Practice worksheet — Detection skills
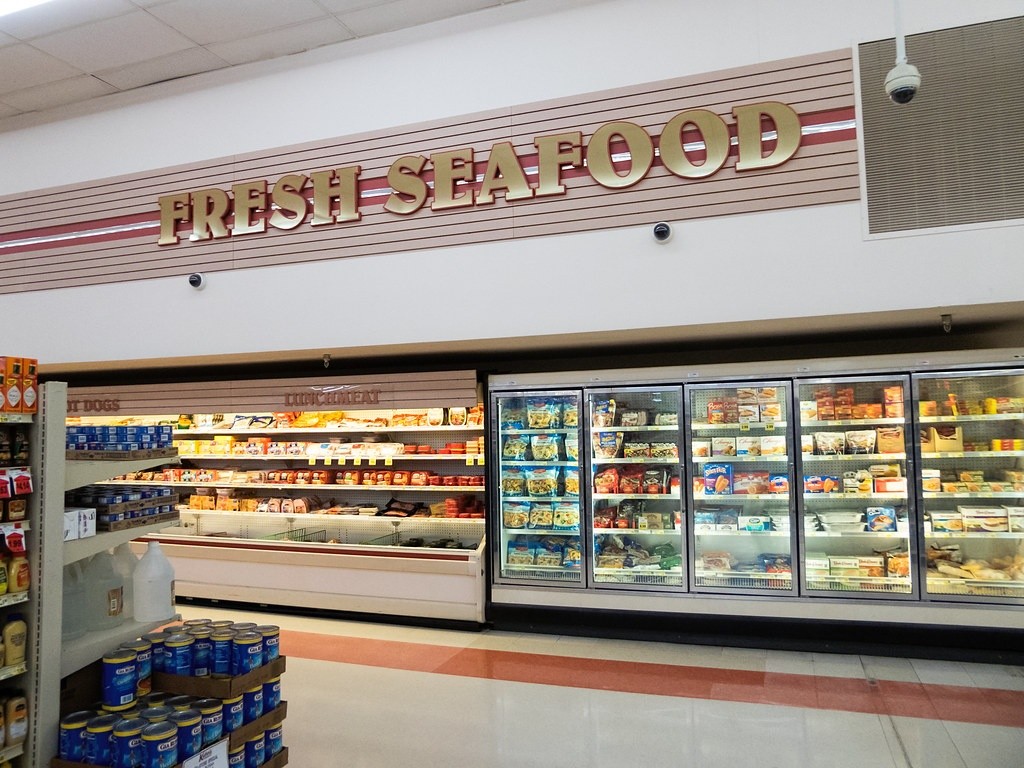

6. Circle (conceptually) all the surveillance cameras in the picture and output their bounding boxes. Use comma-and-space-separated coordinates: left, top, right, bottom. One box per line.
652, 222, 672, 243
188, 272, 206, 290
884, 64, 922, 104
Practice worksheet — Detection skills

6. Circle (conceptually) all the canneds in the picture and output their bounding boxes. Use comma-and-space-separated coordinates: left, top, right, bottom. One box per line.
66, 425, 172, 451
65, 486, 172, 522
60, 619, 283, 768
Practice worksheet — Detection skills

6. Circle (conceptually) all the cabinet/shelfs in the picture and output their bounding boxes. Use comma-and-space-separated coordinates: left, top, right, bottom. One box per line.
0, 381, 183, 768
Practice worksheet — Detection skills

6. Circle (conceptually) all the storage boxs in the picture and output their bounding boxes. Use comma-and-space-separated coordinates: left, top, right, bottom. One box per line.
77, 446, 179, 461
63, 508, 79, 541
95, 510, 180, 531
0, 356, 38, 413
229, 700, 287, 751
163, 468, 180, 482
89, 492, 180, 515
261, 746, 288, 768
150, 653, 287, 701
173, 439, 179, 447
70, 506, 97, 538
64, 424, 174, 450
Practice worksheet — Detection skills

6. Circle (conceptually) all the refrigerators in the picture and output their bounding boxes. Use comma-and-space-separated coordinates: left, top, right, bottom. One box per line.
488, 362, 1024, 611
